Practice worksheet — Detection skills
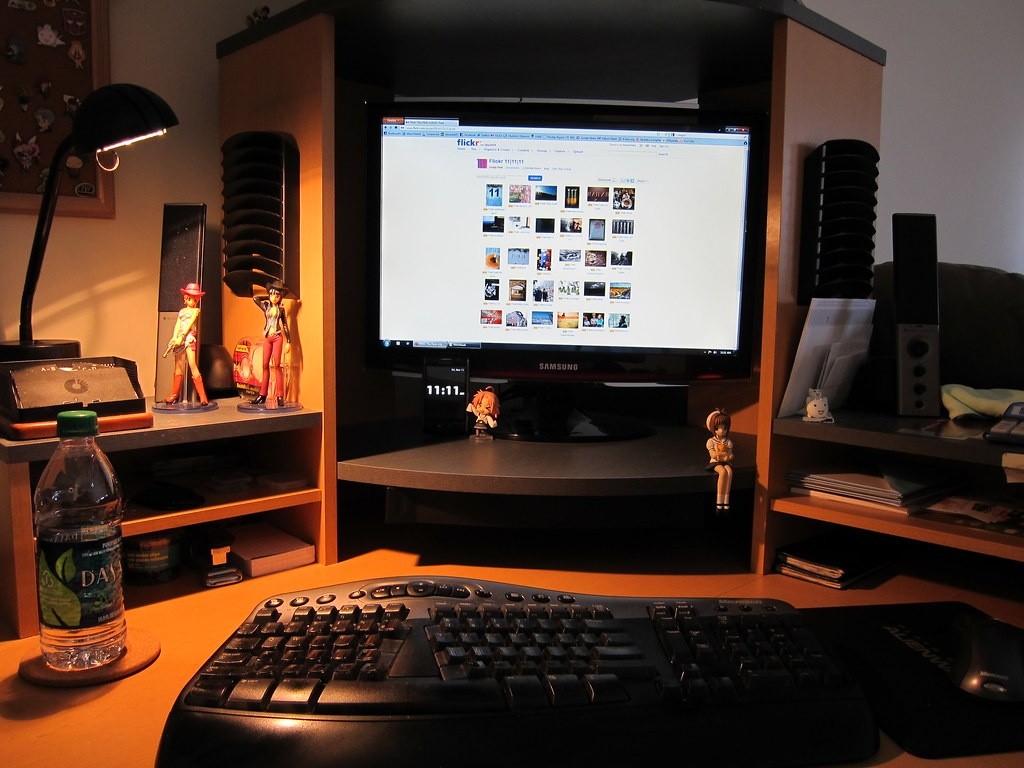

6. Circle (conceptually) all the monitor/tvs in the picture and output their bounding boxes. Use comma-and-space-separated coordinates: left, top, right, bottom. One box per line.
359, 100, 771, 443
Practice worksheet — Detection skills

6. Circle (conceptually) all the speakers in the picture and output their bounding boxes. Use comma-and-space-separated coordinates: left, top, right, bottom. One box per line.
154, 203, 207, 404
893, 213, 941, 418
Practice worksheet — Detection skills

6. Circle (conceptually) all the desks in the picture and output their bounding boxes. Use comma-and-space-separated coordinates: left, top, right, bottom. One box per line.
0, 530, 1024, 768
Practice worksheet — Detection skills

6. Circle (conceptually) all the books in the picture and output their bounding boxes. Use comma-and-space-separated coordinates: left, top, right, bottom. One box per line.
784, 469, 944, 515
773, 536, 893, 589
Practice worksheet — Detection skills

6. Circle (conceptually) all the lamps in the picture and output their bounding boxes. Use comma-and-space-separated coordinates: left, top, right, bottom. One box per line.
0, 80, 178, 362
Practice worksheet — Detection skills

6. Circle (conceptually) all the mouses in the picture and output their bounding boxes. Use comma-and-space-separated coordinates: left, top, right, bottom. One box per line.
939, 659, 1013, 704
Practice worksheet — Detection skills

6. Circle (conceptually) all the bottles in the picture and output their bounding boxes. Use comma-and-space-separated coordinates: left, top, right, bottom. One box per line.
34, 409, 127, 670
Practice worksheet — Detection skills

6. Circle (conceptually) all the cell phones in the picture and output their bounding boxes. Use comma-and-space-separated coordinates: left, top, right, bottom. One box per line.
422, 358, 470, 442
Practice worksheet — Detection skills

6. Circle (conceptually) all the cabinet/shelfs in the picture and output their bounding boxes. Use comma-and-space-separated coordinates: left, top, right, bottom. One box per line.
0, 0, 1024, 633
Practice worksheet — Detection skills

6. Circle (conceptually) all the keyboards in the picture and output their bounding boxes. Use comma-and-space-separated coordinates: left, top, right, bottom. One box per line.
156, 576, 881, 768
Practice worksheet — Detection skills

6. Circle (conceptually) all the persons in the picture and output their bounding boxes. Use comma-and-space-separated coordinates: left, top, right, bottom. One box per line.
249, 285, 291, 407
167, 283, 209, 406
705, 410, 735, 514
465, 391, 499, 437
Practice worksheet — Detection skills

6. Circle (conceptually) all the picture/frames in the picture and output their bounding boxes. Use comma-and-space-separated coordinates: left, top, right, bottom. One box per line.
0, 0, 116, 220
